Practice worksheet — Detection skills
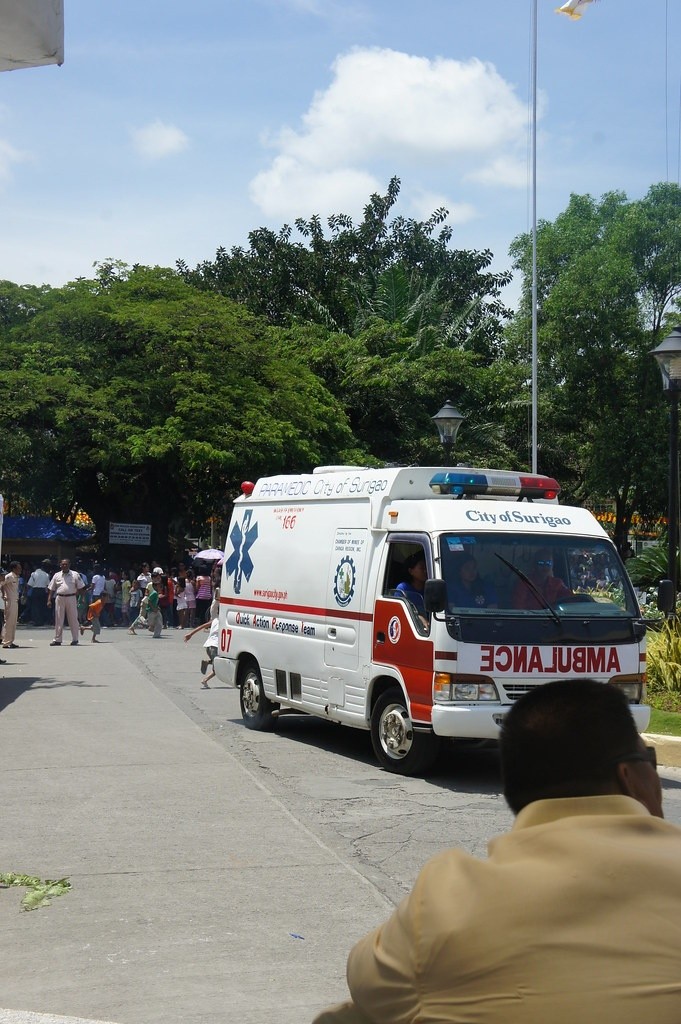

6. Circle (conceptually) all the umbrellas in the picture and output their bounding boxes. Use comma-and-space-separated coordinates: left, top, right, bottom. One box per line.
193, 548, 224, 565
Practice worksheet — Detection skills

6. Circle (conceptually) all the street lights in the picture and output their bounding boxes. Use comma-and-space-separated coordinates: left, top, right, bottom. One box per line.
430, 398, 465, 467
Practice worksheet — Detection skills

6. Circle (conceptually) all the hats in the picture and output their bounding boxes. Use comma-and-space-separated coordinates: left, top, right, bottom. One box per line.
41, 559, 51, 565
172, 577, 180, 585
153, 567, 164, 575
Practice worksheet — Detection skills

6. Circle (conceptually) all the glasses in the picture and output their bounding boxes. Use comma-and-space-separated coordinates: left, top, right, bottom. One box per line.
535, 559, 553, 568
590, 747, 656, 784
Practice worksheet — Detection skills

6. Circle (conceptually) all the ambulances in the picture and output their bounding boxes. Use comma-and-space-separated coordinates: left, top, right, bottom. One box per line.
212, 459, 677, 774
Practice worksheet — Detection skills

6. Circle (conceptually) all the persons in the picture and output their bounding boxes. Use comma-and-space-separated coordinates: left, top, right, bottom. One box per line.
443, 551, 499, 610
390, 549, 430, 631
511, 546, 584, 609
0, 559, 223, 664
344, 678, 680, 1024
183, 605, 220, 690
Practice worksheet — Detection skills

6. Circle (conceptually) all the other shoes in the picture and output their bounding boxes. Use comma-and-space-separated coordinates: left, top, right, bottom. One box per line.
18, 617, 24, 624
175, 625, 184, 629
128, 626, 137, 635
92, 639, 99, 643
162, 626, 167, 629
148, 625, 155, 632
153, 637, 162, 638
0, 660, 6, 664
2, 644, 9, 648
33, 623, 43, 626
8, 643, 19, 648
201, 661, 208, 675
202, 681, 209, 689
50, 641, 61, 646
80, 626, 85, 635
71, 641, 78, 645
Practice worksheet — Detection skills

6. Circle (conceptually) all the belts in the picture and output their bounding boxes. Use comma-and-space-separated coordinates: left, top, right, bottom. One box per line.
33, 588, 45, 590
57, 594, 75, 597
